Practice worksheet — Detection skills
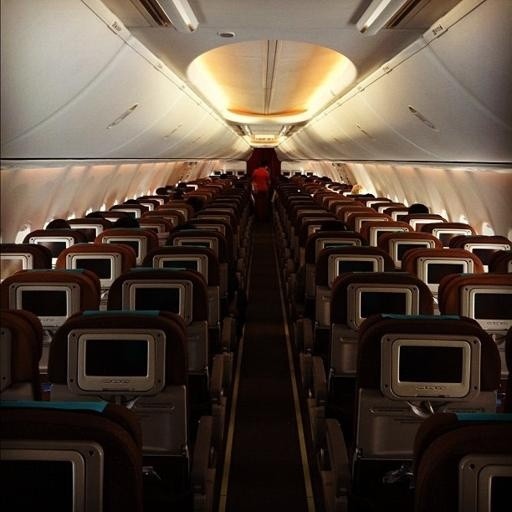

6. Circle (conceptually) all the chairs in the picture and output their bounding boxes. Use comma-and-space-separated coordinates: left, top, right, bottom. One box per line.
272, 174, 512, 510
0, 173, 253, 512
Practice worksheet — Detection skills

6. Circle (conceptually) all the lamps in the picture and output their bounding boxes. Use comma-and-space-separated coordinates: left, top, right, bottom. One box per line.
353, 0, 408, 40
155, 0, 199, 33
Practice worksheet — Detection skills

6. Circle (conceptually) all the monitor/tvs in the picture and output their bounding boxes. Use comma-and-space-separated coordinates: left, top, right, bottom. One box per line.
77, 335, 154, 391
476, 467, 512, 512
294, 200, 512, 328
0, 450, 83, 512
390, 339, 471, 395
213, 169, 245, 177
0, 182, 238, 326
282, 170, 314, 178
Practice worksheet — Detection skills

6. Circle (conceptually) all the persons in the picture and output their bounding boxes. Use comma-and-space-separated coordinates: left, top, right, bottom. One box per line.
250, 160, 272, 217
